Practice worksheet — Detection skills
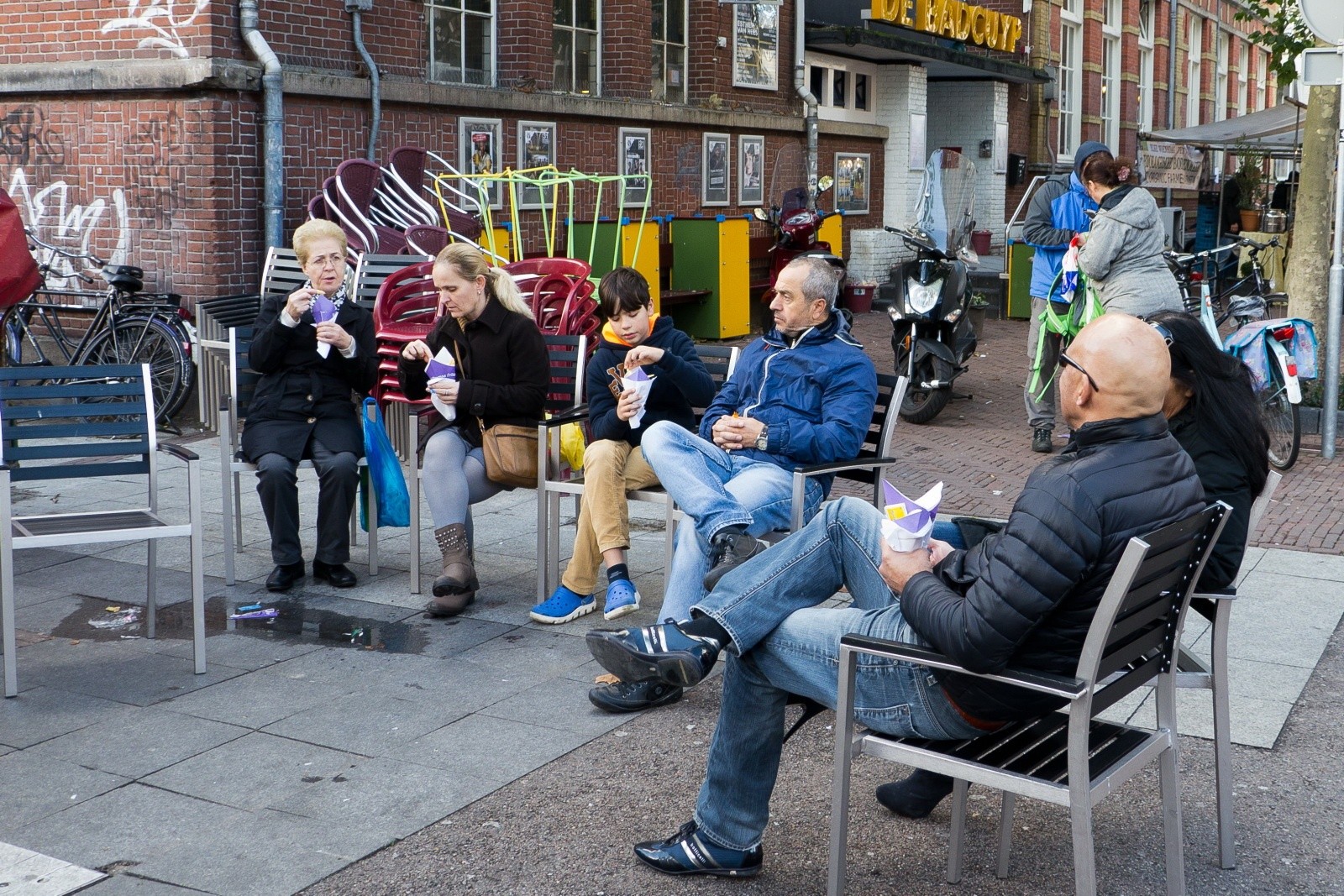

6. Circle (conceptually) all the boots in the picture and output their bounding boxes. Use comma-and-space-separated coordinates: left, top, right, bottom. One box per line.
429, 549, 475, 615
431, 524, 479, 597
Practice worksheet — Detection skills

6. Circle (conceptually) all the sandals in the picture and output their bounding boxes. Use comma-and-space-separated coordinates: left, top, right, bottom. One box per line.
530, 586, 597, 624
604, 579, 641, 620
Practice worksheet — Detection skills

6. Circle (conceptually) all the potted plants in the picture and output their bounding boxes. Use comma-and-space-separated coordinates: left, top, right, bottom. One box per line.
969, 292, 990, 339
1234, 133, 1270, 232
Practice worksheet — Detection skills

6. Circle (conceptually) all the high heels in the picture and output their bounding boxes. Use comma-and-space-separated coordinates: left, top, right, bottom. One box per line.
782, 693, 829, 744
876, 768, 972, 818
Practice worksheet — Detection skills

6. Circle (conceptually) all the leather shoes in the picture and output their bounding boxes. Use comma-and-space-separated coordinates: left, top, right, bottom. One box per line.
265, 556, 305, 591
314, 557, 357, 587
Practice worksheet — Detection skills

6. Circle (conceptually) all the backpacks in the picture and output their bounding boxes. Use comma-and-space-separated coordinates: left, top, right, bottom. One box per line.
1040, 232, 1104, 339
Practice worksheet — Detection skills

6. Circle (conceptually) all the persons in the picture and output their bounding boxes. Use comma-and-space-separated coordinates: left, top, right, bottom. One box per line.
711, 144, 724, 169
849, 167, 864, 201
627, 159, 644, 185
1221, 166, 1300, 292
242, 218, 380, 592
587, 255, 879, 713
1022, 141, 1187, 452
529, 267, 717, 625
744, 143, 759, 188
472, 133, 492, 174
526, 158, 549, 180
587, 307, 1272, 876
398, 243, 551, 618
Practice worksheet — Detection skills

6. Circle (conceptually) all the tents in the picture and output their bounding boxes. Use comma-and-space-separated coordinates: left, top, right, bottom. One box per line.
1137, 101, 1308, 294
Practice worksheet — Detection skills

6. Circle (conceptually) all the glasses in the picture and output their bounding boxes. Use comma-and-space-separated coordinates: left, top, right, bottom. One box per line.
305, 254, 346, 266
1137, 315, 1175, 349
1059, 347, 1099, 392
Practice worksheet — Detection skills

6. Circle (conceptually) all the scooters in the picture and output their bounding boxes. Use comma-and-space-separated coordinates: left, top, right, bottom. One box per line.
882, 147, 982, 426
753, 141, 848, 335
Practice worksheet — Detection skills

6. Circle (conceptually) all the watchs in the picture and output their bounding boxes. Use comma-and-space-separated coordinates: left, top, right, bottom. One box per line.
755, 425, 767, 451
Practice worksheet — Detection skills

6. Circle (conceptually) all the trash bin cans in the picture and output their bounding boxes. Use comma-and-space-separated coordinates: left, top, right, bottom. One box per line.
846, 284, 876, 314
973, 230, 993, 255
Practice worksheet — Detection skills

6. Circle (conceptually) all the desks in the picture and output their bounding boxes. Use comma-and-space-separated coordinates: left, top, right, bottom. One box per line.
1237, 230, 1288, 293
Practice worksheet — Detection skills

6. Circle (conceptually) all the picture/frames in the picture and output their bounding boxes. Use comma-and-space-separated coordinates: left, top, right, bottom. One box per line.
909, 111, 927, 170
732, 0, 779, 90
617, 127, 651, 208
737, 135, 764, 206
458, 117, 502, 210
833, 152, 870, 215
701, 132, 730, 207
516, 120, 556, 209
994, 122, 1009, 173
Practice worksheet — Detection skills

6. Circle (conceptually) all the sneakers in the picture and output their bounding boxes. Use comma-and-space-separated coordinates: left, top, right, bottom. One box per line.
703, 532, 767, 592
586, 617, 720, 687
1032, 428, 1053, 453
633, 821, 763, 878
589, 680, 683, 714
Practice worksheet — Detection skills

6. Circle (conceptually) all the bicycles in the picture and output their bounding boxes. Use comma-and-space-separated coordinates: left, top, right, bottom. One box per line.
1160, 231, 1303, 471
0, 223, 199, 440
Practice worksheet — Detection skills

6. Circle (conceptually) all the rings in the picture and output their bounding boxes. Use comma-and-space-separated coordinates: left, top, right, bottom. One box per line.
446, 389, 448, 395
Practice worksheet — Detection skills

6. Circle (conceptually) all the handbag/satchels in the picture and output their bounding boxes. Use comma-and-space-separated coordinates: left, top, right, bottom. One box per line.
1221, 318, 1318, 392
1060, 233, 1080, 305
360, 397, 410, 532
547, 422, 587, 470
0, 189, 43, 309
483, 423, 552, 488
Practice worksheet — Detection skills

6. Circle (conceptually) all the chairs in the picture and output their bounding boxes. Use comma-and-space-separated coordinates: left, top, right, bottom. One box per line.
195, 146, 601, 600
828, 499, 1235, 896
1, 363, 206, 698
664, 374, 910, 597
537, 345, 741, 604
996, 470, 1283, 878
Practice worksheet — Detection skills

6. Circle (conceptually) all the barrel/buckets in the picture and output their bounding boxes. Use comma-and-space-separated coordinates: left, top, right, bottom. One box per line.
1260, 208, 1287, 234
845, 283, 876, 314
970, 230, 994, 256
